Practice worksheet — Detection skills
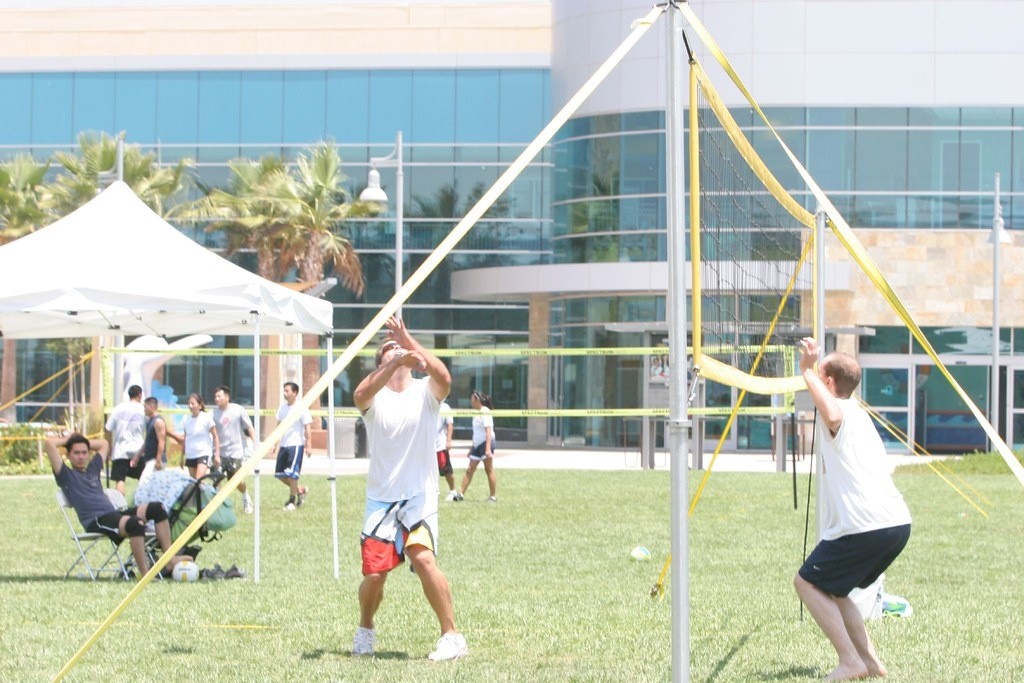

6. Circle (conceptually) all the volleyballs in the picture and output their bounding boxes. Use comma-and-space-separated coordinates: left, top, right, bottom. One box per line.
630, 546, 652, 565
172, 561, 199, 583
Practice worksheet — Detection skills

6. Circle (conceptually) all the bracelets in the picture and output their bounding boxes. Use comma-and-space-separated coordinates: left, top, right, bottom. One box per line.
173, 434, 176, 439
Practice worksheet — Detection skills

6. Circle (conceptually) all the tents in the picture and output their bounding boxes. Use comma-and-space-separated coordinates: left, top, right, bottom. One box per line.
0, 180, 340, 583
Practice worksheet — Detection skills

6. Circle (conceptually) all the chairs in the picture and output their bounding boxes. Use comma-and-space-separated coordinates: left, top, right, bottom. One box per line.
56, 488, 163, 582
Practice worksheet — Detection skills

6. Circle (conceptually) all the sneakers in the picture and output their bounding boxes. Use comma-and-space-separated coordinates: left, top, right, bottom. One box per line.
354, 627, 375, 658
285, 495, 295, 506
241, 496, 254, 515
428, 633, 468, 661
296, 487, 308, 506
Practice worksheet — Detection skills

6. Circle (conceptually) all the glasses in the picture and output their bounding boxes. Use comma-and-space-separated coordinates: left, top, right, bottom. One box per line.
381, 341, 404, 357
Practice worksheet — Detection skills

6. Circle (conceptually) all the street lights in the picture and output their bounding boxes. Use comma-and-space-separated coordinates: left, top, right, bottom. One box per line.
359, 129, 408, 342
983, 170, 1012, 457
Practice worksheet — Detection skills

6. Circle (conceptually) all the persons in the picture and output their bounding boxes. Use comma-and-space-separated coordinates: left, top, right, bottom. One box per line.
353, 315, 467, 662
213, 385, 256, 514
44, 432, 192, 582
437, 403, 457, 502
270, 382, 312, 511
105, 385, 184, 497
794, 337, 913, 681
129, 397, 167, 506
453, 391, 499, 504
180, 394, 221, 484
650, 355, 693, 380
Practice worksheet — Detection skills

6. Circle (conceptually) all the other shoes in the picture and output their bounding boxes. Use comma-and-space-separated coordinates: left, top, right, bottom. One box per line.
453, 493, 464, 501
484, 496, 497, 503
199, 563, 247, 580
281, 502, 296, 511
446, 489, 457, 502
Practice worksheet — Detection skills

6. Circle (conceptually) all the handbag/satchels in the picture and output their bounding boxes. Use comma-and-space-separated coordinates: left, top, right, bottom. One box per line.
198, 483, 236, 533
